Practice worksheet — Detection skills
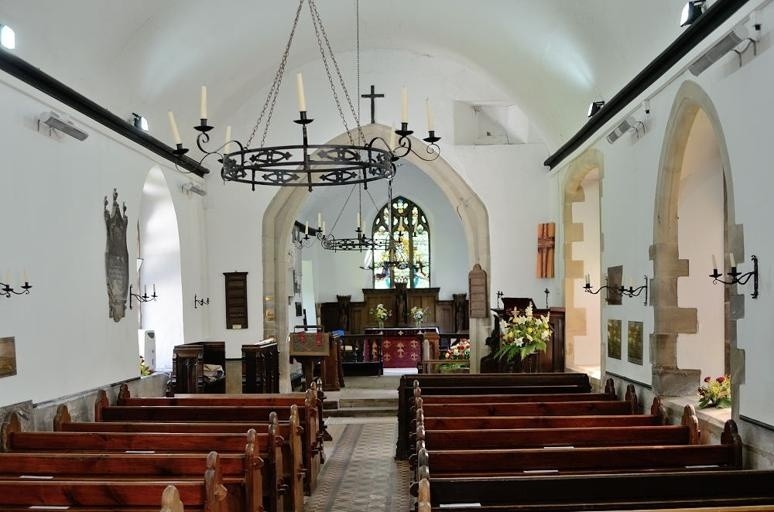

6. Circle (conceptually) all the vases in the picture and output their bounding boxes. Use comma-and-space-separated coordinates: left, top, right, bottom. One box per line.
415, 318, 422, 328
378, 320, 383, 327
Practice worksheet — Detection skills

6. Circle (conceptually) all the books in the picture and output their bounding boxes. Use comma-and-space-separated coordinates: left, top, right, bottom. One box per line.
330, 330, 344, 337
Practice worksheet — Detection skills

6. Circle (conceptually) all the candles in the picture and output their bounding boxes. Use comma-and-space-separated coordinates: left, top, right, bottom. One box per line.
585, 273, 590, 283
712, 255, 717, 269
4, 269, 28, 285
629, 277, 633, 286
622, 277, 624, 286
144, 284, 155, 293
729, 253, 735, 267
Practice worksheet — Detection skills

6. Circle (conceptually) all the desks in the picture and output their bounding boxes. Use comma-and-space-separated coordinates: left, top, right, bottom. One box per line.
364, 327, 439, 368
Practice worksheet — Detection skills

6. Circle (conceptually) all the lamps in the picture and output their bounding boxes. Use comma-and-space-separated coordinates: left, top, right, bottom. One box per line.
679, 0, 705, 27
33, 111, 89, 141
134, 0, 441, 280
607, 116, 650, 145
686, 25, 760, 79
587, 101, 604, 117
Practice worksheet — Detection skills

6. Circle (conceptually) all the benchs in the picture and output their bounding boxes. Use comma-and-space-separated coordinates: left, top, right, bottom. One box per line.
0, 377, 333, 512
395, 372, 774, 512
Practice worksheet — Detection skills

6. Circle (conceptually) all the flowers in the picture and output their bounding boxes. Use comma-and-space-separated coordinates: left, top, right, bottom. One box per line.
494, 301, 552, 363
408, 305, 431, 322
370, 303, 392, 321
441, 338, 470, 368
